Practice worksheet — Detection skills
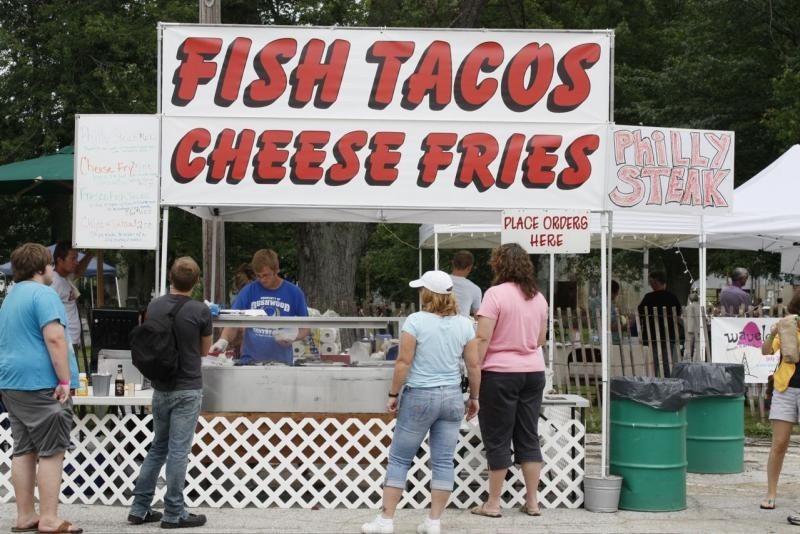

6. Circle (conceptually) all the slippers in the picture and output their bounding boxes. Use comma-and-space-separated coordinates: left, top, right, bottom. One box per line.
760, 500, 774, 509
13, 521, 39, 532
520, 501, 541, 515
471, 502, 502, 517
35, 520, 82, 534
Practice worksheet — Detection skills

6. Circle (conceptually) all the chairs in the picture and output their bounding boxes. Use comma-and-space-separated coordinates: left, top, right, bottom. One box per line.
566, 348, 602, 407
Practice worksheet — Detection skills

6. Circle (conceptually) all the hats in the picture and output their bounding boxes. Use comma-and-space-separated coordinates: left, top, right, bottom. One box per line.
409, 270, 453, 294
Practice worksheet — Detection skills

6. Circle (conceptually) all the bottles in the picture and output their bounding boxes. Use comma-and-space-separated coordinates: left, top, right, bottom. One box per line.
77, 372, 88, 396
114, 364, 125, 396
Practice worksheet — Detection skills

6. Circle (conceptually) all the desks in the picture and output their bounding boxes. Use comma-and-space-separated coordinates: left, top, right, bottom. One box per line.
540, 394, 591, 508
70, 387, 155, 496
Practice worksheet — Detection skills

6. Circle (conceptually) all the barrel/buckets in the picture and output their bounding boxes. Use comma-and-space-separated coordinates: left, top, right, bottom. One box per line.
583, 474, 623, 512
91, 374, 112, 397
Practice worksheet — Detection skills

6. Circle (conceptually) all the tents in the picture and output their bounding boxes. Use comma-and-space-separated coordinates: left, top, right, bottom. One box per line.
0, 243, 122, 310
674, 144, 800, 317
157, 205, 607, 479
420, 212, 710, 361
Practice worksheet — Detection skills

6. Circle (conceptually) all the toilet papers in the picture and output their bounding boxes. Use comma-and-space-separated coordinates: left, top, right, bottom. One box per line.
293, 344, 305, 357
320, 327, 338, 343
321, 342, 342, 356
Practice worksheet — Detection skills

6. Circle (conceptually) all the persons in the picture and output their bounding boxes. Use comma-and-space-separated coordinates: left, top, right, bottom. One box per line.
638, 269, 685, 378
592, 279, 624, 345
207, 248, 311, 366
361, 269, 481, 534
760, 289, 800, 525
0, 243, 83, 534
475, 242, 549, 518
719, 266, 752, 314
448, 250, 482, 320
48, 241, 81, 373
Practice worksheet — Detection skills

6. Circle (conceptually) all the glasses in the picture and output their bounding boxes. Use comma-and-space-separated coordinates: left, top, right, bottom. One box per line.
255, 271, 273, 280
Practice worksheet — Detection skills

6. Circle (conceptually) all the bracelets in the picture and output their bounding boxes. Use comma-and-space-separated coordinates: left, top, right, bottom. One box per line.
469, 395, 479, 400
58, 380, 70, 385
389, 391, 398, 397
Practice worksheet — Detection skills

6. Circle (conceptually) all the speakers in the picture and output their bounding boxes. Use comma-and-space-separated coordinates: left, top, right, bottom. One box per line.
91, 307, 148, 384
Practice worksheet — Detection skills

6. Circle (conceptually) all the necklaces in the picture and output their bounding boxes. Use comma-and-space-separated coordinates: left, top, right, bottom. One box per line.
128, 255, 213, 529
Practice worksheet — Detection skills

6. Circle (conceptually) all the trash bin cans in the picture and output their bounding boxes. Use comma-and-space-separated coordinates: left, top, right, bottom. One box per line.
672, 362, 745, 474
609, 376, 688, 512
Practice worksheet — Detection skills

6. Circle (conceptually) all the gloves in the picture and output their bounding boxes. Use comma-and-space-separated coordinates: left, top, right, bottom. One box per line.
209, 338, 229, 354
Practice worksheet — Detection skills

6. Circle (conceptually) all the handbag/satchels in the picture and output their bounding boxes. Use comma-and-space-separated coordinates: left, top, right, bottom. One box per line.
129, 313, 176, 381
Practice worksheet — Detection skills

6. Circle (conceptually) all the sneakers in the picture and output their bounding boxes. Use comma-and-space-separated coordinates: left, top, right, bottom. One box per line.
362, 515, 393, 534
417, 517, 440, 534
128, 511, 162, 523
161, 514, 206, 528
787, 514, 800, 524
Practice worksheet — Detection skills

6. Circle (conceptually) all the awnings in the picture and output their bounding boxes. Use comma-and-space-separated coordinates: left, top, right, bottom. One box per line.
0, 143, 73, 180
